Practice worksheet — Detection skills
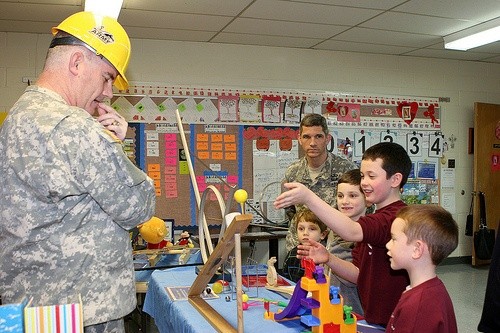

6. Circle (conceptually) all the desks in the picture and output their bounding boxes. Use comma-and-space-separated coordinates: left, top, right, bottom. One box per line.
194, 231, 289, 269
129, 247, 203, 333
142, 264, 386, 333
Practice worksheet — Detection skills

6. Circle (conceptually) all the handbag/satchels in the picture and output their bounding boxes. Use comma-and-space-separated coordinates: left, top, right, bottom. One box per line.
474, 228, 495, 261
465, 215, 473, 236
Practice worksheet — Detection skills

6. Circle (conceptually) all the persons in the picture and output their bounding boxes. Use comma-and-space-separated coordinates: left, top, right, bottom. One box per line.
273, 114, 460, 333
0, 10, 157, 332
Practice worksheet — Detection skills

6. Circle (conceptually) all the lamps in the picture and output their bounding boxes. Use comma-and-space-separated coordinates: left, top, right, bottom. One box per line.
443, 16, 500, 51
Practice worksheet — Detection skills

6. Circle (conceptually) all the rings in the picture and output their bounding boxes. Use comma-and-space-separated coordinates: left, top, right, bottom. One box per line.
113, 120, 120, 126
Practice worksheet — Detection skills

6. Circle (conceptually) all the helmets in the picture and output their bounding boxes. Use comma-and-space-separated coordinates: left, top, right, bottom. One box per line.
51, 11, 131, 91
